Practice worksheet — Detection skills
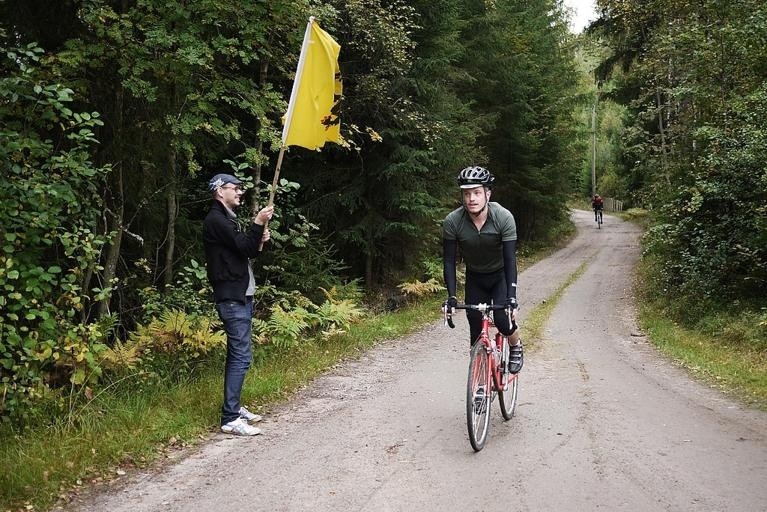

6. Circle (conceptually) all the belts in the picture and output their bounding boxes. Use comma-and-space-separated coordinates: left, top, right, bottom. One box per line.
225, 295, 253, 305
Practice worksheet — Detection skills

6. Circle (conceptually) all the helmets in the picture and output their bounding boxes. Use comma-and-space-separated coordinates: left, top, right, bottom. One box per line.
455, 164, 495, 194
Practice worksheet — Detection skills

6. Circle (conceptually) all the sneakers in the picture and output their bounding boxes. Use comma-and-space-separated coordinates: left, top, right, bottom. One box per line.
471, 388, 487, 414
506, 337, 525, 373
239, 406, 261, 422
221, 417, 261, 437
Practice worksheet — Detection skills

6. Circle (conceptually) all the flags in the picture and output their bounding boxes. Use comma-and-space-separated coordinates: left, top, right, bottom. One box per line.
283, 23, 348, 150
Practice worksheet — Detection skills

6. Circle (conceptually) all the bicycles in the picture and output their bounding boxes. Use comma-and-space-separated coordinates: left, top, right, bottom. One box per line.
593, 208, 603, 229
442, 299, 521, 451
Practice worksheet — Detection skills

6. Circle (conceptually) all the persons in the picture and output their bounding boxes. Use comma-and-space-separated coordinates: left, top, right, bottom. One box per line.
593, 194, 603, 223
441, 163, 521, 373
203, 172, 274, 437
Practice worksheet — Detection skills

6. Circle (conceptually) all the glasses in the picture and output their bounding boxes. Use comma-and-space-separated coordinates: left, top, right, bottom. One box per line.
222, 186, 241, 192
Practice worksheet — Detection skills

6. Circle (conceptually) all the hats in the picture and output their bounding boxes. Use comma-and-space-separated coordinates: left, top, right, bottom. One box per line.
207, 171, 244, 192
